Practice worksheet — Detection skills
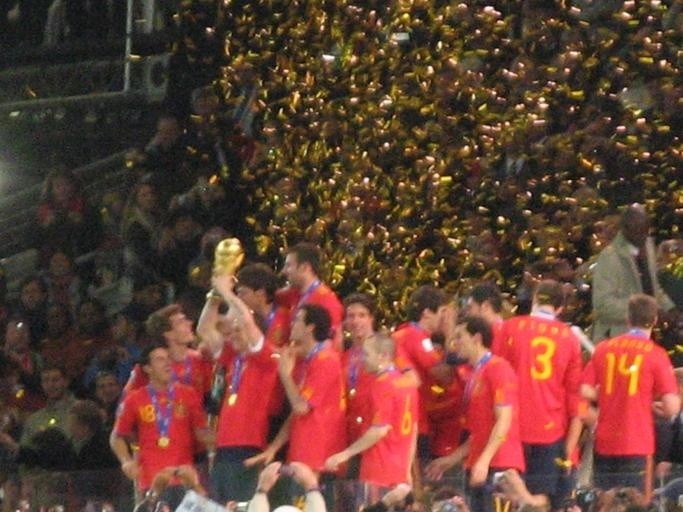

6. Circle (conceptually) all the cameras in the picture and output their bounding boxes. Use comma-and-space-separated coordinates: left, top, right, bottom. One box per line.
406, 492, 414, 503
575, 490, 594, 507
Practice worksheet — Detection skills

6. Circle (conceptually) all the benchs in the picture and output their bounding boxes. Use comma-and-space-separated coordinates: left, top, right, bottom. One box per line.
0, 148, 139, 303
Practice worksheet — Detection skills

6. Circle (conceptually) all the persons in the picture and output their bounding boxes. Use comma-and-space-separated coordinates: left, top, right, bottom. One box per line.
0, 1, 683, 512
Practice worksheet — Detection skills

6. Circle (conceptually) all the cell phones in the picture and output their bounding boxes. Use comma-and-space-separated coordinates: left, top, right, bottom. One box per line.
492, 473, 506, 486
279, 466, 294, 477
